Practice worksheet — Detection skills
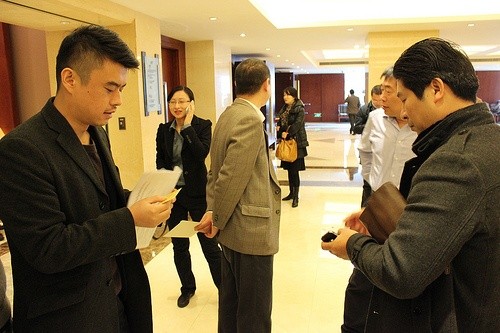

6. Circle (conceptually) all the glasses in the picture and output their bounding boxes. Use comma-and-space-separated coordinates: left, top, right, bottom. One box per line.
169, 100, 191, 105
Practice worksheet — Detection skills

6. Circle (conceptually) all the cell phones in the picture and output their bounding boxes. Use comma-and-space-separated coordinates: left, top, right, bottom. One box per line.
185, 100, 194, 116
321, 232, 337, 242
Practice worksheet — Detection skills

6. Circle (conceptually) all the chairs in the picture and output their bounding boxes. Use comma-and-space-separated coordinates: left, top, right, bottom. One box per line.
338, 104, 349, 123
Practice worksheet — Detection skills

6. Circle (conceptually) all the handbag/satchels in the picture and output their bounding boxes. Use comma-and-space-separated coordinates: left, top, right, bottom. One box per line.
275, 124, 298, 163
358, 181, 409, 244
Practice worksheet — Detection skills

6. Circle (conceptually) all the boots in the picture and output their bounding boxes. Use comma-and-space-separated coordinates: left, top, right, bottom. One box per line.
282, 186, 295, 200
291, 186, 299, 208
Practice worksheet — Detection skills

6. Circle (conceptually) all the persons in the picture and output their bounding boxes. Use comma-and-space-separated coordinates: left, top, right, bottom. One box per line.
276, 86, 309, 207
358, 66, 419, 197
321, 38, 500, 333
0, 24, 177, 333
344, 89, 360, 135
193, 58, 281, 332
352, 85, 383, 209
155, 85, 221, 307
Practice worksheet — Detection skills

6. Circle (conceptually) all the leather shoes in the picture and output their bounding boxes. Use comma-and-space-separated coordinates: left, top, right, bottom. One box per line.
177, 289, 195, 308
